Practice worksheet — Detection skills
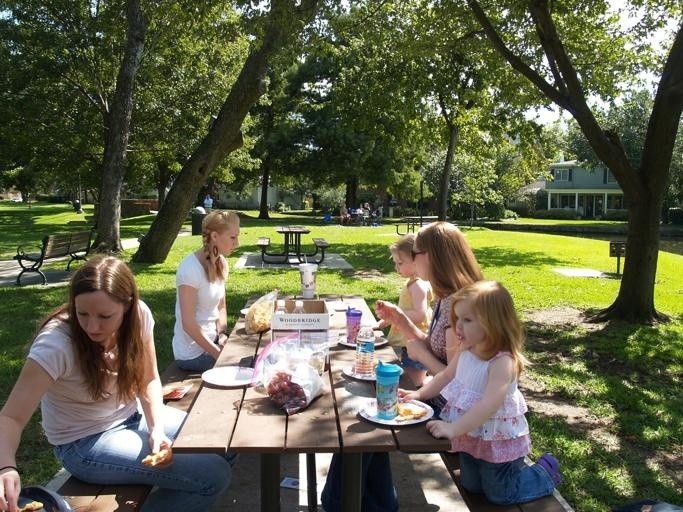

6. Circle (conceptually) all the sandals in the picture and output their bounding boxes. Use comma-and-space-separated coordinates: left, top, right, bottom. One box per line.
537, 451, 561, 488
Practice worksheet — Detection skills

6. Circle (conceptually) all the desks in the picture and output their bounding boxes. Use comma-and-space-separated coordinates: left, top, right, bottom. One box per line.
337, 217, 356, 225
276, 226, 311, 265
351, 213, 370, 225
405, 215, 450, 237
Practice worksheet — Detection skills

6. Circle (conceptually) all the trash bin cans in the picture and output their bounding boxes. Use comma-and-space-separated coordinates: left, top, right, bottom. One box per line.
192, 214, 206, 235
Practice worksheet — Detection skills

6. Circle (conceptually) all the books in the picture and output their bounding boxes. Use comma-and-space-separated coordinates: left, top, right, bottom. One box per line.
162, 378, 194, 400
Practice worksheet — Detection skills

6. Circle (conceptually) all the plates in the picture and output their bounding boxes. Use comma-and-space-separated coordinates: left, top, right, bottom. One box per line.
201, 366, 255, 387
343, 363, 405, 382
339, 337, 388, 348
359, 397, 435, 427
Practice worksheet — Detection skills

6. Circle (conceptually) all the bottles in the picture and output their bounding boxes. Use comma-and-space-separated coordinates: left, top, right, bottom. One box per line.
276, 299, 307, 315
355, 323, 375, 376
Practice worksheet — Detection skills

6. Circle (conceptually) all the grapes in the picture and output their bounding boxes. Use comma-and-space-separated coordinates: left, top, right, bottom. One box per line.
268, 371, 309, 413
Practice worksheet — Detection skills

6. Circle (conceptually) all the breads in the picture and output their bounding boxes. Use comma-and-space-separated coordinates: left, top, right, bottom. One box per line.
398, 401, 427, 421
20, 501, 43, 512
141, 450, 167, 467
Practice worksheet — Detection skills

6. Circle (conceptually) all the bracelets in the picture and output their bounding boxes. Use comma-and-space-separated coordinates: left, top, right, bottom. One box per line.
0, 466, 19, 471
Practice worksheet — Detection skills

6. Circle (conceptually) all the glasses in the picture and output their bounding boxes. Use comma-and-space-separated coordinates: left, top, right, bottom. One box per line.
410, 250, 426, 262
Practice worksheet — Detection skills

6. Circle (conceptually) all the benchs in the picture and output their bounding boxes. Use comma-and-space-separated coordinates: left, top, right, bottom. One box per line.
310, 238, 330, 264
44, 358, 205, 511
257, 237, 276, 264
13, 230, 93, 286
394, 223, 405, 235
388, 451, 581, 512
361, 219, 374, 225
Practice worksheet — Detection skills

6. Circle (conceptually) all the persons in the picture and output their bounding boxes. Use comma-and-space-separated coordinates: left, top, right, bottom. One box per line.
0, 256, 241, 512
320, 200, 384, 226
195, 194, 214, 214
172, 209, 239, 372
397, 279, 561, 507
320, 221, 484, 512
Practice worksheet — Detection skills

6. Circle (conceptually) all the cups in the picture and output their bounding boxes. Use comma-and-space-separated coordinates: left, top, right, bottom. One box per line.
345, 306, 362, 343
374, 362, 402, 420
298, 262, 318, 298
